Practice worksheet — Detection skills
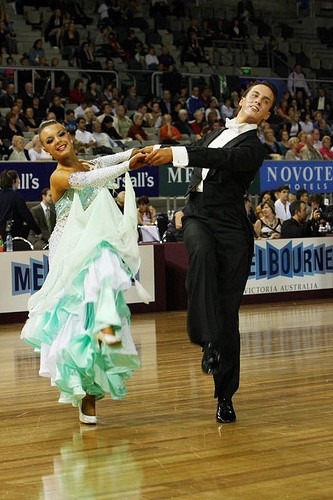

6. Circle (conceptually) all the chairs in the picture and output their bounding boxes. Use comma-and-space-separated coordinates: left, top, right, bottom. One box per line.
0, 0, 333, 163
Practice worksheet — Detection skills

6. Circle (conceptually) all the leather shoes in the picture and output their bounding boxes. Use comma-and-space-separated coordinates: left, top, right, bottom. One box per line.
201, 341, 222, 373
216, 395, 236, 424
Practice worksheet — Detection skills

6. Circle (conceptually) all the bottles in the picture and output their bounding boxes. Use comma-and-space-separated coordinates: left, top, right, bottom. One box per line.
325, 222, 331, 232
0, 235, 4, 252
7, 233, 13, 252
143, 213, 147, 227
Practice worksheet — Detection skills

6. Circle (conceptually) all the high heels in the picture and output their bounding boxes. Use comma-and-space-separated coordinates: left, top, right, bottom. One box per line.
78, 400, 98, 423
97, 331, 122, 353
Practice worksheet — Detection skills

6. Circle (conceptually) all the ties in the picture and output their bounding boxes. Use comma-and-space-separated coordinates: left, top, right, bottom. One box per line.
47, 210, 51, 232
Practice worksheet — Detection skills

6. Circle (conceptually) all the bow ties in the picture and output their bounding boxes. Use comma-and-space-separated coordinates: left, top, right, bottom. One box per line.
224, 118, 239, 134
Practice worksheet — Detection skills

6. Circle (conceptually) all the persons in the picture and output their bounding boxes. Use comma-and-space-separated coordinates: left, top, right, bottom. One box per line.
138, 81, 277, 421
107, 187, 187, 241
38, 118, 151, 423
1, 0, 333, 161
243, 183, 333, 238
0, 168, 58, 250
